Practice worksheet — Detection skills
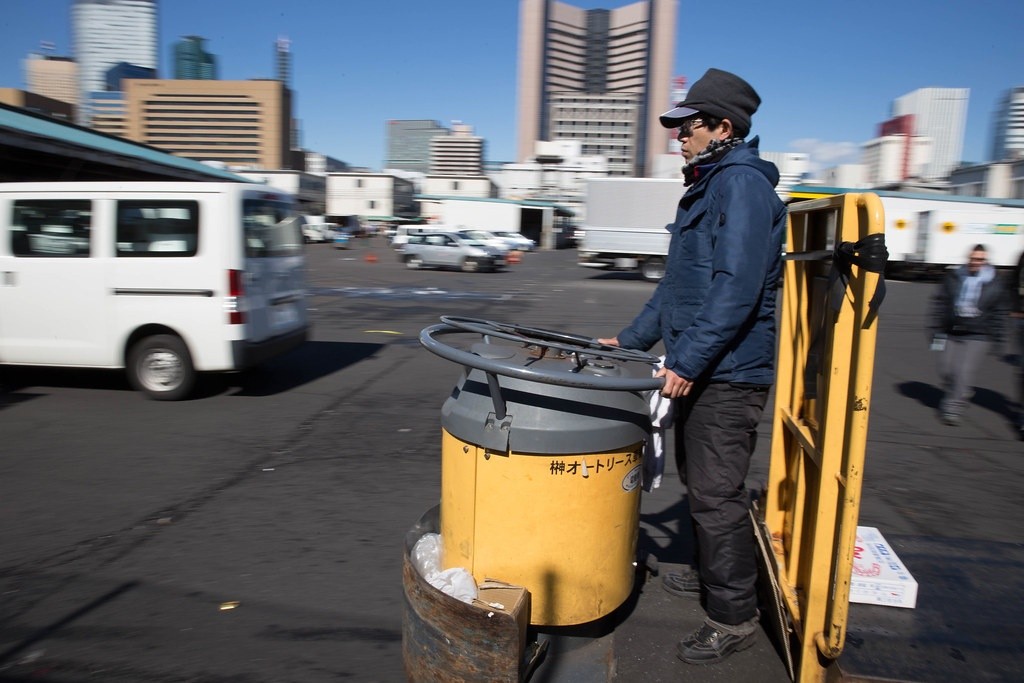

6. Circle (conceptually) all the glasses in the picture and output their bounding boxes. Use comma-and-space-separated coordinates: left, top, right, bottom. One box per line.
679, 117, 705, 134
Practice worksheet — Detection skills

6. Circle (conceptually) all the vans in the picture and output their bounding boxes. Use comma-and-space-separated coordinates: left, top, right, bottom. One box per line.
0, 182, 314, 401
302, 221, 341, 246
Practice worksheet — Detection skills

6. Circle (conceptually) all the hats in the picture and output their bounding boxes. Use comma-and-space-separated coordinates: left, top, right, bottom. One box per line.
658, 67, 760, 138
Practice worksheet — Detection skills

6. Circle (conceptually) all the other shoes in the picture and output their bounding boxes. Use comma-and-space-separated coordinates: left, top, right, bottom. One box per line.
942, 411, 961, 426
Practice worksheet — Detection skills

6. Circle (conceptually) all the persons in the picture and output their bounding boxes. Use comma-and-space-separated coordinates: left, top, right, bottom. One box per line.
597, 68, 787, 666
923, 244, 1014, 426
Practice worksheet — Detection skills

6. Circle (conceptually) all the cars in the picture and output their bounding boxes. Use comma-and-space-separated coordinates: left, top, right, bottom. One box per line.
389, 221, 535, 276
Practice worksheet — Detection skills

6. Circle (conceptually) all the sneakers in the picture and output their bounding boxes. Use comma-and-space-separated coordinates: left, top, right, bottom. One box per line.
662, 570, 701, 599
677, 614, 757, 663
